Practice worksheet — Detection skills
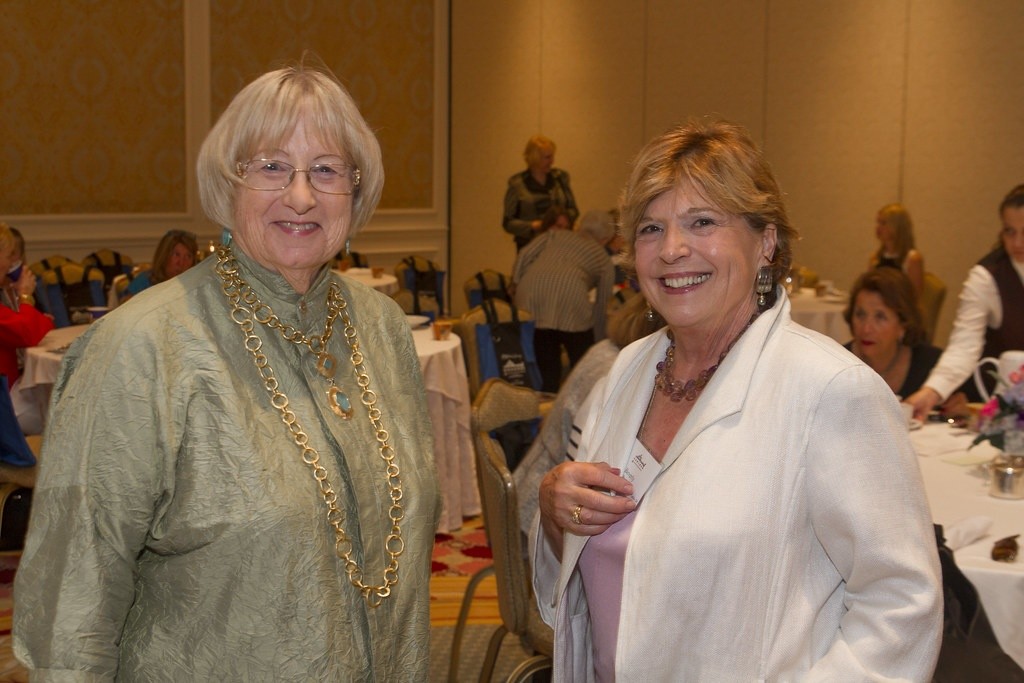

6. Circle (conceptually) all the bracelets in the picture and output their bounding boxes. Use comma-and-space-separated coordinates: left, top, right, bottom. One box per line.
19, 294, 36, 306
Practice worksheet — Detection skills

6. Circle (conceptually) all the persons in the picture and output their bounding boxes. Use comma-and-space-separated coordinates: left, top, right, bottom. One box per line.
11, 68, 442, 683
904, 184, 1024, 422
127, 229, 199, 299
841, 266, 975, 418
507, 209, 616, 394
527, 122, 944, 683
502, 136, 578, 252
511, 291, 666, 538
0, 223, 54, 390
875, 203, 925, 291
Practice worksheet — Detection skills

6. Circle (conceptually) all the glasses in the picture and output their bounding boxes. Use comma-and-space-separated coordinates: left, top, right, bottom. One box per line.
236, 159, 363, 194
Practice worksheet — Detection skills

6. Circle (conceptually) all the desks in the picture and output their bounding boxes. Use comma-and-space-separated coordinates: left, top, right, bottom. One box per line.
905, 420, 1024, 671
17, 313, 481, 537
786, 281, 855, 349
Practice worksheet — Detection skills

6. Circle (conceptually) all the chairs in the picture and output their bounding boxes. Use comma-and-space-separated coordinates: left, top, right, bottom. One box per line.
24, 249, 155, 330
336, 249, 363, 271
920, 272, 946, 343
397, 255, 615, 683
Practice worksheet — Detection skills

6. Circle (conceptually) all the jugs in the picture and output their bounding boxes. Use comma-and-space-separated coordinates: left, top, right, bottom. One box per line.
974, 349, 1024, 403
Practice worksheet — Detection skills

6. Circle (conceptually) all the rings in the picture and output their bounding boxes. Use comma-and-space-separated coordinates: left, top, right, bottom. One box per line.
571, 505, 583, 523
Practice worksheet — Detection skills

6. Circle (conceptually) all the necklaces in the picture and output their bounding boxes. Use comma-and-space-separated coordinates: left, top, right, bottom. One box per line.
215, 245, 405, 609
862, 346, 901, 379
655, 314, 758, 402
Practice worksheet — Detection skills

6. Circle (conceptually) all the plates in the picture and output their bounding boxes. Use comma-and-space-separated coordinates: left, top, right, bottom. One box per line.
909, 420, 923, 431
406, 316, 430, 328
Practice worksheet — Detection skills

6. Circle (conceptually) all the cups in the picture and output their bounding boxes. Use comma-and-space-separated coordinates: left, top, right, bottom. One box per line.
88, 307, 110, 323
969, 403, 985, 431
432, 322, 452, 341
7, 260, 22, 282
372, 267, 384, 280
898, 402, 912, 424
991, 464, 1024, 501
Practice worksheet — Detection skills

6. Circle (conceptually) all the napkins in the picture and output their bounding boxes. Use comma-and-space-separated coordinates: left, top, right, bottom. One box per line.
942, 515, 990, 550
